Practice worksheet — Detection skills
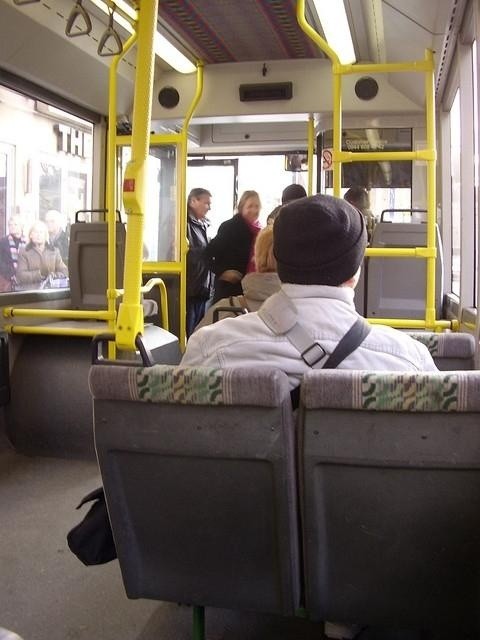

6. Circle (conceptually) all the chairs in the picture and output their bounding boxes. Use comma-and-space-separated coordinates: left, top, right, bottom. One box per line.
299, 367, 479, 639
89, 361, 299, 638
65, 207, 160, 317
367, 204, 447, 321
413, 334, 479, 367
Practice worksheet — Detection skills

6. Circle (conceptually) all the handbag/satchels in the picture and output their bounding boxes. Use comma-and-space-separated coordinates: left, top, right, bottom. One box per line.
67, 488, 117, 565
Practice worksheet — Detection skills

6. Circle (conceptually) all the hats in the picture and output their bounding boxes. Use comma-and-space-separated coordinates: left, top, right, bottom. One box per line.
273, 194, 368, 286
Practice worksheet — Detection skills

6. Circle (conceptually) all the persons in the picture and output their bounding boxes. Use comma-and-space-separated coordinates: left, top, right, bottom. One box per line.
1, 208, 69, 293
176, 195, 440, 372
168, 184, 377, 344
